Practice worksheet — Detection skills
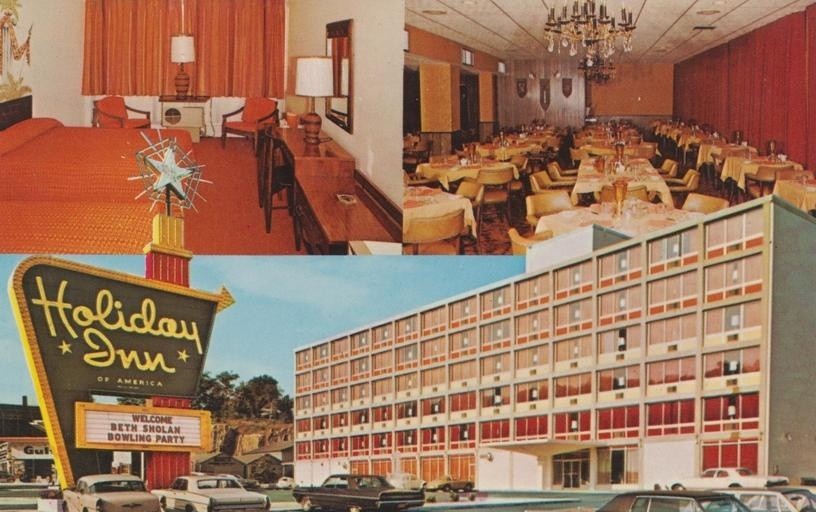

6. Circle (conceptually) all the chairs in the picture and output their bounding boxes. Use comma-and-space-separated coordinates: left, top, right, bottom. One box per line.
221, 95, 279, 156
91, 94, 151, 128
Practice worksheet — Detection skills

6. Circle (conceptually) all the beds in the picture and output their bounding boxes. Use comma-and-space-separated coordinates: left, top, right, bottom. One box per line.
0, 94, 195, 254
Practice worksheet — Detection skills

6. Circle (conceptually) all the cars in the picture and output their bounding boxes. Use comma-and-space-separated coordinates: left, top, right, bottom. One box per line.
62, 474, 162, 511
595, 490, 752, 512
386, 474, 427, 489
710, 489, 816, 512
665, 467, 790, 491
151, 474, 271, 511
426, 476, 475, 492
292, 474, 426, 511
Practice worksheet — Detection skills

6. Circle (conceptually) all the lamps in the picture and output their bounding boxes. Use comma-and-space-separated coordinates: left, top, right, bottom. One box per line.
169, 32, 196, 101
540, 0, 637, 84
293, 55, 334, 143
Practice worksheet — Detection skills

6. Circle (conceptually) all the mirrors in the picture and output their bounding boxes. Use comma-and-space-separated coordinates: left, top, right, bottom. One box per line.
324, 18, 353, 133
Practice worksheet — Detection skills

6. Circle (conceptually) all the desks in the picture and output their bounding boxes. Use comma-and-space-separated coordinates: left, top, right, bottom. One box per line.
159, 94, 211, 143
259, 121, 402, 255
